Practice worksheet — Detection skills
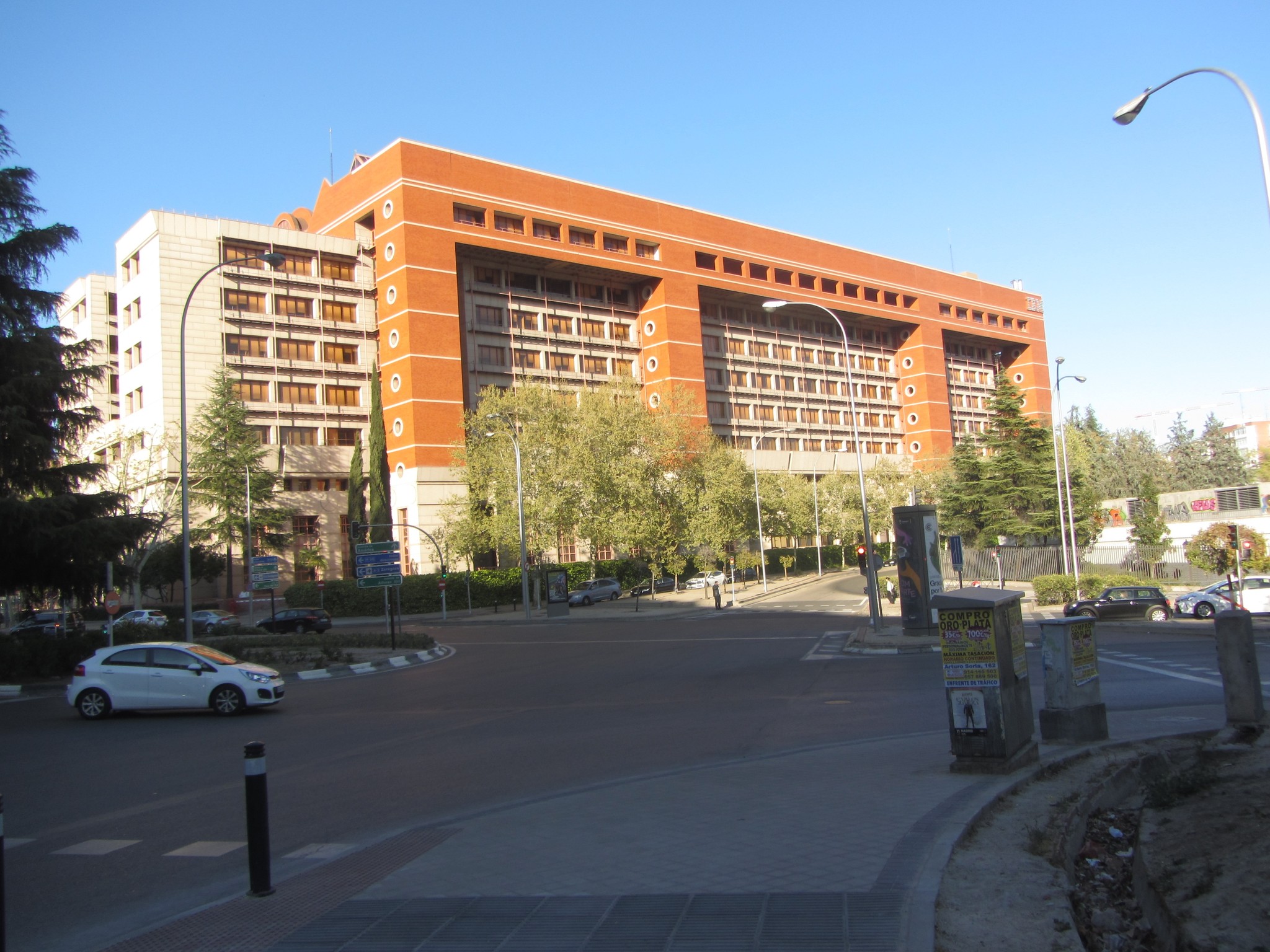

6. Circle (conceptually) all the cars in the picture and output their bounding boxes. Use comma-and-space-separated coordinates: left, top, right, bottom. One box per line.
177, 609, 241, 634
256, 607, 331, 634
66, 641, 285, 721
686, 571, 728, 589
112, 610, 171, 629
883, 553, 897, 567
1174, 575, 1270, 620
725, 568, 757, 583
630, 577, 680, 597
567, 578, 622, 607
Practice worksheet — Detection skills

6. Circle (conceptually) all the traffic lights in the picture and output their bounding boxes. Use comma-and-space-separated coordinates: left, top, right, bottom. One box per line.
350, 520, 360, 539
1241, 540, 1253, 562
441, 565, 447, 579
729, 556, 734, 569
992, 545, 1001, 562
857, 547, 866, 568
1227, 525, 1239, 550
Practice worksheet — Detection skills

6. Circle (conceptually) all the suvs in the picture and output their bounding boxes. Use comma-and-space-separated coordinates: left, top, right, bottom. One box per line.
8, 611, 85, 635
1062, 587, 1173, 622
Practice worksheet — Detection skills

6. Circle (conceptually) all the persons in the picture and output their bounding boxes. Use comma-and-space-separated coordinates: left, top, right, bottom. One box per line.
886, 577, 895, 604
1110, 590, 1128, 600
964, 700, 975, 728
713, 581, 723, 610
1233, 582, 1240, 590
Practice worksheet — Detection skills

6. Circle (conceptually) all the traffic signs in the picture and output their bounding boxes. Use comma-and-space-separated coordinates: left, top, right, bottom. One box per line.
356, 541, 402, 589
250, 556, 280, 590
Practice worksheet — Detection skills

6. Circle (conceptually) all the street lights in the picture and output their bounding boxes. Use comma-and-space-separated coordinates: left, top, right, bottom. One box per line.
813, 448, 847, 577
762, 300, 880, 634
1052, 356, 1086, 606
485, 413, 531, 622
212, 441, 253, 624
755, 429, 798, 592
1114, 68, 1270, 199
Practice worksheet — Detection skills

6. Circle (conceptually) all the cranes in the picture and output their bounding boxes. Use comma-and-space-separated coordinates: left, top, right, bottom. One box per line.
1134, 388, 1270, 438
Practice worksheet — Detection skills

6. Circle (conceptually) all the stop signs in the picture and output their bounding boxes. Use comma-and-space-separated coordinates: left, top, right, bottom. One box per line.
316, 580, 326, 590
438, 580, 446, 590
